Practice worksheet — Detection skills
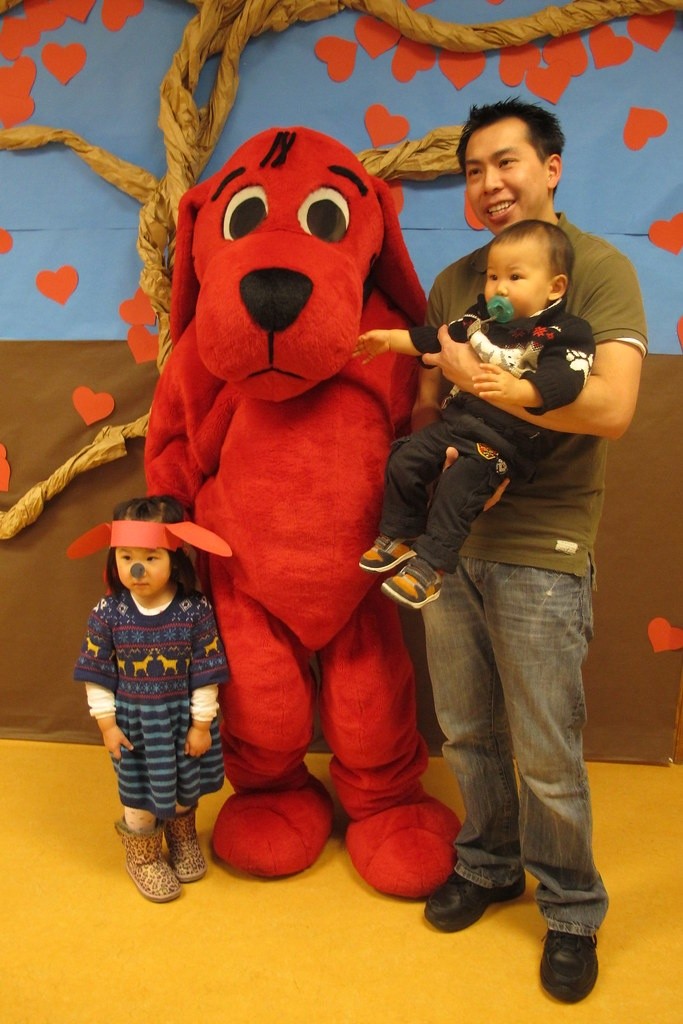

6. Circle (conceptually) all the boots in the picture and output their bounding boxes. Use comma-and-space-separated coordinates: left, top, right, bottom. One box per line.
114, 812, 181, 903
166, 799, 206, 882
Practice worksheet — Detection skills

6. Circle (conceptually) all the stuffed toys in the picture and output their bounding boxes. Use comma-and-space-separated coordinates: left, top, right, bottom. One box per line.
141, 125, 463, 902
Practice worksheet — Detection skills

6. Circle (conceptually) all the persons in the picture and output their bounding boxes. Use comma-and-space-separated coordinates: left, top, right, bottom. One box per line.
413, 94, 649, 1004
353, 219, 597, 609
65, 494, 233, 903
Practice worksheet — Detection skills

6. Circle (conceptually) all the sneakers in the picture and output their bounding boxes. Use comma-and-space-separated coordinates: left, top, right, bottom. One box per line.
358, 531, 423, 573
539, 925, 599, 1003
381, 555, 445, 610
425, 869, 526, 933
